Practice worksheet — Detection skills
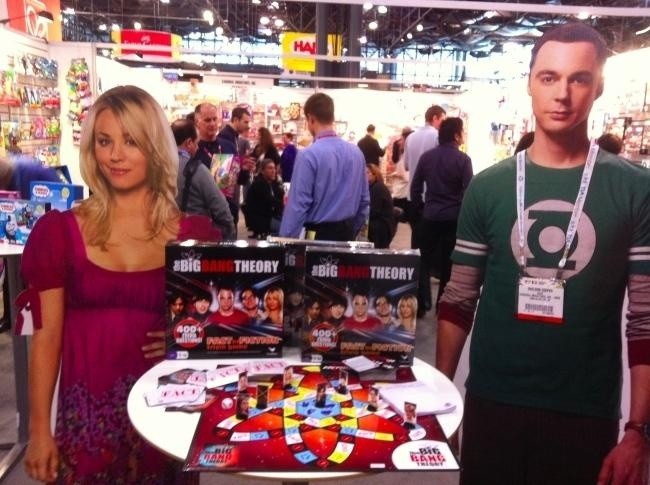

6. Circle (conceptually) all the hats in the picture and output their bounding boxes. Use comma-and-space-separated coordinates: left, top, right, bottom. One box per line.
193, 289, 212, 303
328, 295, 348, 308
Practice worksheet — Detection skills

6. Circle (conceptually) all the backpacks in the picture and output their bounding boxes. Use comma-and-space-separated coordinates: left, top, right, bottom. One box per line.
171, 205, 225, 244
390, 139, 401, 163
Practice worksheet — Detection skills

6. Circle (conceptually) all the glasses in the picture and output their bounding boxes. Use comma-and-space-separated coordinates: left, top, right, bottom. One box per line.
354, 300, 367, 306
219, 295, 232, 300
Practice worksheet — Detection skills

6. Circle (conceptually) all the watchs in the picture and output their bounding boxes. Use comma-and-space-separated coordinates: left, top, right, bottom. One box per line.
624, 420, 650, 441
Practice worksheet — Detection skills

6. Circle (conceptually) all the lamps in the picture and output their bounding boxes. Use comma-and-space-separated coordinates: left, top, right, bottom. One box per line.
0, 9, 55, 27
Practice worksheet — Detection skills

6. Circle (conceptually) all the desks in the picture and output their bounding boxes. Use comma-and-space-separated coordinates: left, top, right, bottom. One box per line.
0, 239, 29, 484
122, 349, 468, 485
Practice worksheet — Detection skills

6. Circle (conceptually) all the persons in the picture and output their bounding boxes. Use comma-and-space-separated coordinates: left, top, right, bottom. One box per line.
435, 19, 650, 482
283, 367, 292, 388
596, 132, 625, 156
514, 132, 535, 157
172, 103, 297, 239
404, 105, 473, 300
357, 123, 415, 249
338, 371, 347, 391
280, 94, 370, 242
367, 386, 380, 411
169, 281, 416, 331
237, 372, 248, 391
20, 82, 221, 483
236, 394, 248, 418
0, 150, 68, 197
317, 384, 325, 406
401, 403, 415, 428
